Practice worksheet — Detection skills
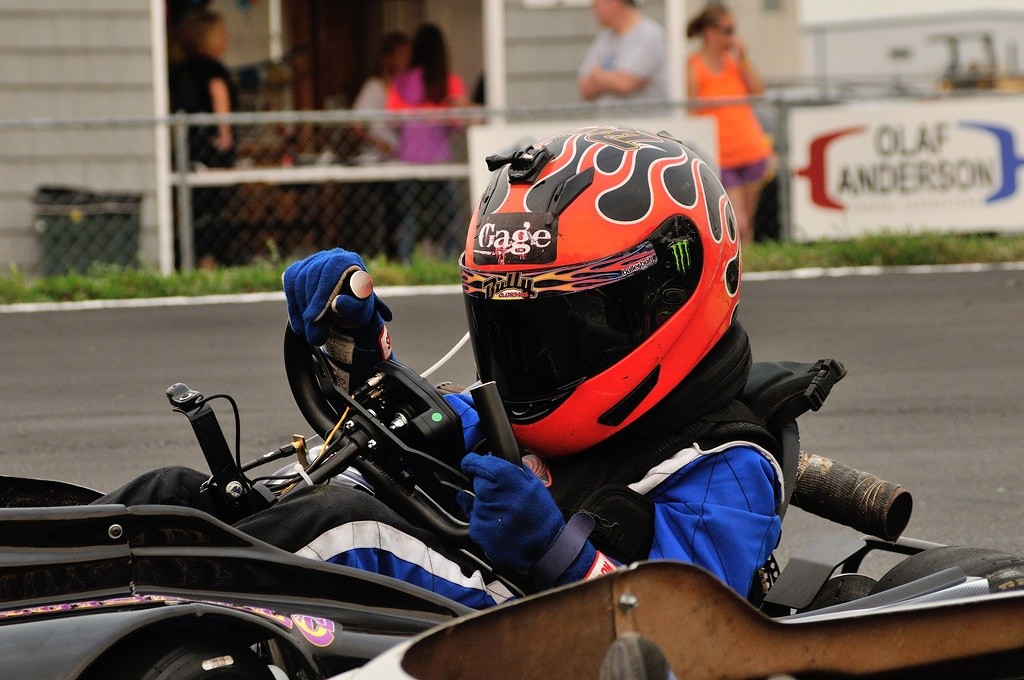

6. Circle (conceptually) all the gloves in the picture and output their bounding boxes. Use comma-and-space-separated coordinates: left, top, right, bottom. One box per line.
277, 246, 395, 359
459, 451, 612, 591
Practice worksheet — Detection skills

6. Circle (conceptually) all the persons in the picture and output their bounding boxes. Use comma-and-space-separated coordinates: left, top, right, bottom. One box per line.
352, 21, 467, 268
169, 8, 238, 271
686, 0, 774, 247
578, 0, 669, 124
282, 126, 787, 611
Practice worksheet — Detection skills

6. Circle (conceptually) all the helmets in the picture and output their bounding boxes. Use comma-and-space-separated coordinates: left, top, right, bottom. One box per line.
459, 126, 742, 449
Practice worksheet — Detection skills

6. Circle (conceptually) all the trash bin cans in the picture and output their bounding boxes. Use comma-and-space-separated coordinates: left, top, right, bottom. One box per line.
33, 183, 142, 282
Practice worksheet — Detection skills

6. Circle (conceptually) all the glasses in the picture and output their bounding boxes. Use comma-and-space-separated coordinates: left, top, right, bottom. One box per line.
710, 24, 734, 36
